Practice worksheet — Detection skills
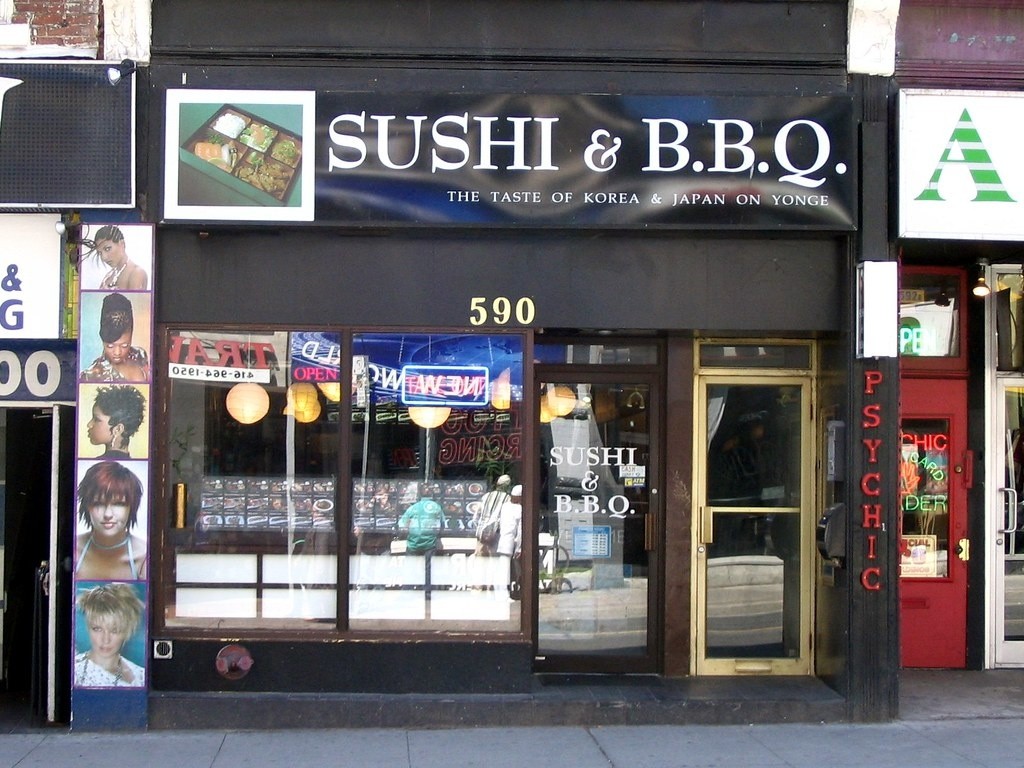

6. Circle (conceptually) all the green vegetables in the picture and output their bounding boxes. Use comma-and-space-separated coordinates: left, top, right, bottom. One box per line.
204, 124, 297, 166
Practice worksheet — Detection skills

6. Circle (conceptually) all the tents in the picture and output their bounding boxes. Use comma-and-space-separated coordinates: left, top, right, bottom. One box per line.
288, 378, 341, 424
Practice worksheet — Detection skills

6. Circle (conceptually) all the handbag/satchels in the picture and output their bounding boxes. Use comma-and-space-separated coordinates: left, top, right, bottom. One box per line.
480, 521, 500, 545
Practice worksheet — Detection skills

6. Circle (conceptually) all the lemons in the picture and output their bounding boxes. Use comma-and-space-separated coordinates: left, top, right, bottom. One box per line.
208, 158, 225, 165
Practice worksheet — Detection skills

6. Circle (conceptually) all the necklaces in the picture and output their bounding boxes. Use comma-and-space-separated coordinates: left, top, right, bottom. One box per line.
81, 651, 122, 686
109, 258, 129, 287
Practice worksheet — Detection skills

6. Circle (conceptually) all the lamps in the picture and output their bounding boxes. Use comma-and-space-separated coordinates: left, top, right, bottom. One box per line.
106, 65, 137, 85
972, 269, 990, 296
285, 335, 576, 428
225, 334, 269, 425
55, 222, 80, 235
934, 287, 950, 307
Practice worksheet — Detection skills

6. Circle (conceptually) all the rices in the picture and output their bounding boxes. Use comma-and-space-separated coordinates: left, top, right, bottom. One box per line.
213, 113, 246, 139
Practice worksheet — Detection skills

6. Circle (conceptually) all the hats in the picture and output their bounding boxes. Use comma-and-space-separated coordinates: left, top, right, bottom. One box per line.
496, 474, 511, 490
511, 485, 523, 496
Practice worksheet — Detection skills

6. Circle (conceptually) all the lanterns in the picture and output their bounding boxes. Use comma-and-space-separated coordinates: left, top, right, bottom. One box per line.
491, 358, 577, 424
224, 381, 270, 426
408, 405, 453, 428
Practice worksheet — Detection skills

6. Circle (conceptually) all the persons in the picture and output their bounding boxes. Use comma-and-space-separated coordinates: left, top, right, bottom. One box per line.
77, 461, 144, 581
715, 425, 783, 555
397, 484, 444, 557
87, 386, 146, 459
81, 293, 147, 382
94, 226, 148, 292
474, 475, 523, 602
74, 582, 145, 686
